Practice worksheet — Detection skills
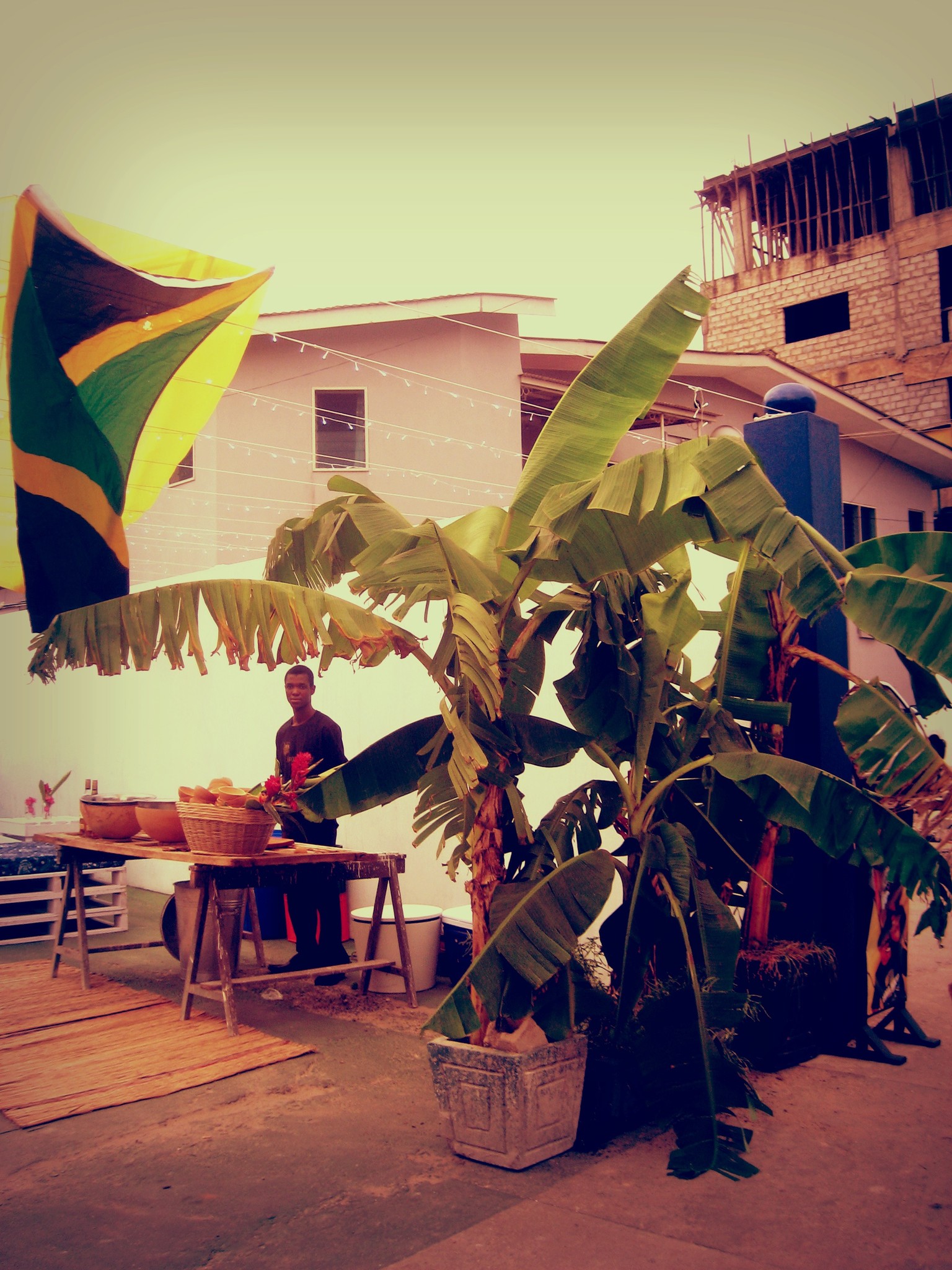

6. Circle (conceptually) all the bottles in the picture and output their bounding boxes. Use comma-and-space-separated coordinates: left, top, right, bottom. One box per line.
80, 779, 98, 838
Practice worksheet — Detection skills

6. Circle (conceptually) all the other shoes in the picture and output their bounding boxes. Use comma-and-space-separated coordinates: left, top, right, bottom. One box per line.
268, 951, 315, 974
314, 944, 350, 987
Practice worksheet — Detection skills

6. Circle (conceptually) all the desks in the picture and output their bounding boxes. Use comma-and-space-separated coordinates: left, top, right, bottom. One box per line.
0, 840, 128, 945
0, 815, 80, 843
34, 832, 418, 1036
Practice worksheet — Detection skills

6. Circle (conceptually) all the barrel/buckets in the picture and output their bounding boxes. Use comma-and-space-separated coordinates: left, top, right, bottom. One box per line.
173, 880, 251, 983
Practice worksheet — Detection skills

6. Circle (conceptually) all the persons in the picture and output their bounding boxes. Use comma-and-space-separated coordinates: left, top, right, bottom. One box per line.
268, 665, 352, 986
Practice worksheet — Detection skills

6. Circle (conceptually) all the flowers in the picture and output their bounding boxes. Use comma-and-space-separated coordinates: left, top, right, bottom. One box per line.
24, 769, 71, 819
256, 751, 324, 825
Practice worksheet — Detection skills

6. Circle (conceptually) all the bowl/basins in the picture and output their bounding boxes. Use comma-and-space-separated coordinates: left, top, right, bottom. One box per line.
134, 799, 186, 843
178, 777, 265, 809
161, 892, 179, 962
81, 793, 156, 839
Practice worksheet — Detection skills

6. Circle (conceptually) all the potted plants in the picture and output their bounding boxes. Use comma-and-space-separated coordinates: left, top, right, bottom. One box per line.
28, 267, 952, 1184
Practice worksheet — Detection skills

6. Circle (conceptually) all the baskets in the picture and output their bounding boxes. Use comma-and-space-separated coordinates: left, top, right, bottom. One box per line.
176, 801, 277, 856
